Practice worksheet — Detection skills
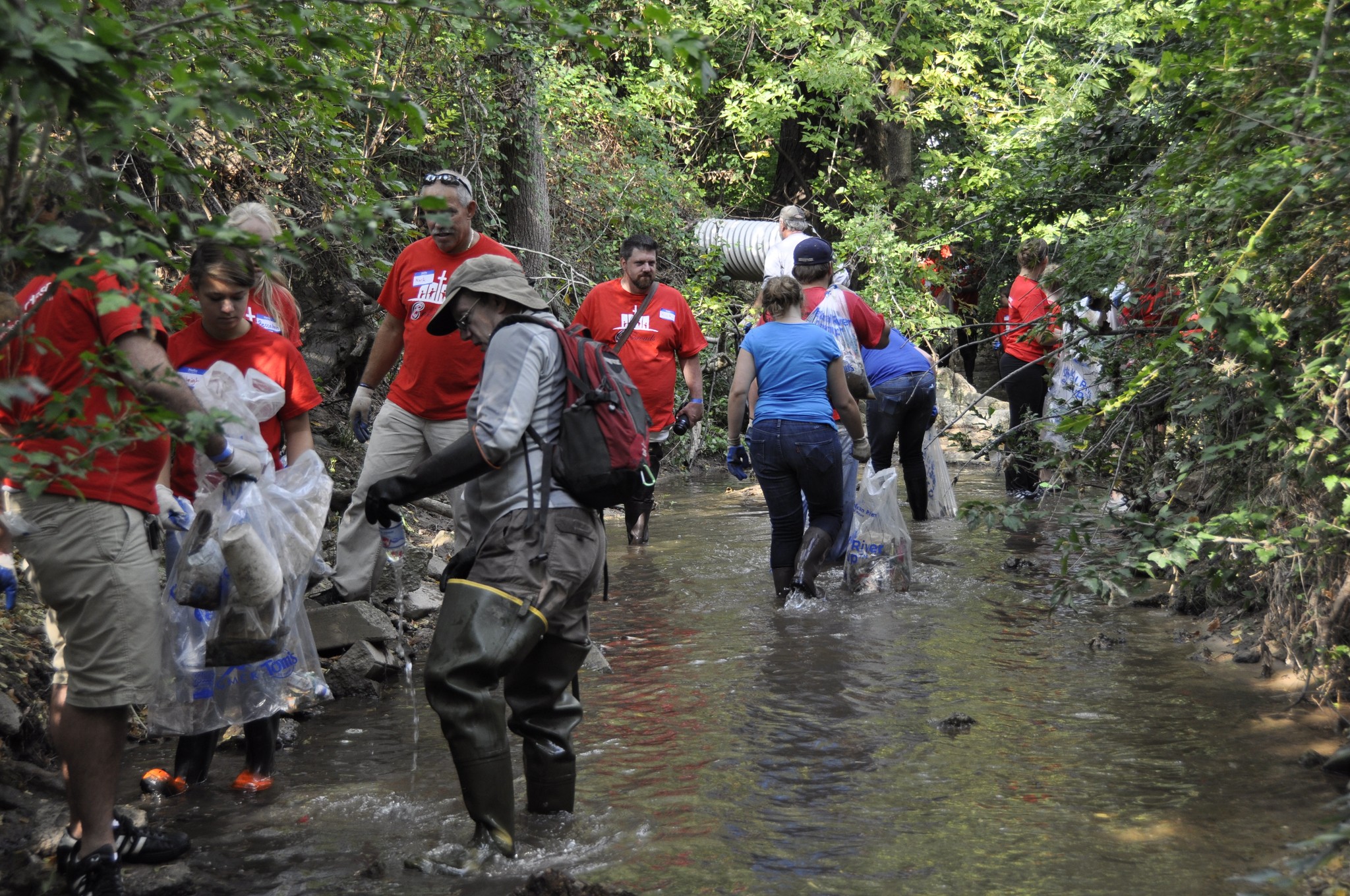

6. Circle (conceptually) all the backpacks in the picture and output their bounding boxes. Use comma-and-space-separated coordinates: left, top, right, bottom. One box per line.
488, 315, 655, 509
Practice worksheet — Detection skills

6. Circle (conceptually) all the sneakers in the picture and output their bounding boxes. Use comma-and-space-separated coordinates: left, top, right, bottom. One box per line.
64, 835, 126, 896
56, 809, 192, 875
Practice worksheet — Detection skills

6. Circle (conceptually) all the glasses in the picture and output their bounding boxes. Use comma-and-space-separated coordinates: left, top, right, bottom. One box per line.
242, 243, 280, 253
456, 297, 481, 331
422, 173, 472, 198
1000, 295, 1007, 298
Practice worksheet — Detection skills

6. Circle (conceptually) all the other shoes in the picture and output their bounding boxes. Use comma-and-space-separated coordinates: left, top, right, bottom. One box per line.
230, 765, 280, 793
1101, 493, 1129, 516
308, 586, 346, 606
140, 768, 207, 797
1005, 486, 1050, 501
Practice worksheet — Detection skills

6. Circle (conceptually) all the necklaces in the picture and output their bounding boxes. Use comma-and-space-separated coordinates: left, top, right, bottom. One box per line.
460, 228, 477, 255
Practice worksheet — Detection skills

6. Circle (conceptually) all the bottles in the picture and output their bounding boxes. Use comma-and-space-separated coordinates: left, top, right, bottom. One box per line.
287, 671, 332, 699
377, 504, 407, 570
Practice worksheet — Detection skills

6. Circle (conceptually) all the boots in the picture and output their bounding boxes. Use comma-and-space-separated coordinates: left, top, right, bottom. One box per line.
771, 566, 794, 599
623, 498, 656, 545
522, 753, 578, 813
401, 751, 517, 881
793, 526, 832, 599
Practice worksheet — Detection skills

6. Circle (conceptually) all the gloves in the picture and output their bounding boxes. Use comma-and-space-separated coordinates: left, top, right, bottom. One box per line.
726, 436, 750, 481
364, 424, 505, 529
216, 448, 262, 482
850, 432, 872, 463
992, 339, 1001, 349
155, 483, 190, 531
439, 542, 476, 593
0, 551, 18, 610
349, 384, 375, 444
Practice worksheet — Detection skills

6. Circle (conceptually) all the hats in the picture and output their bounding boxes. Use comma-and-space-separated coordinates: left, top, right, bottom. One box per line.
779, 205, 806, 221
426, 254, 551, 336
793, 237, 837, 264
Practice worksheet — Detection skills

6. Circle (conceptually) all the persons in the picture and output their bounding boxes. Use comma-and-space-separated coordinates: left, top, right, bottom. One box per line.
736, 204, 850, 333
727, 276, 872, 610
1109, 253, 1222, 522
1037, 262, 1069, 424
857, 323, 938, 519
998, 236, 1064, 501
156, 201, 304, 361
745, 237, 890, 564
905, 241, 987, 389
316, 171, 524, 605
988, 287, 1014, 392
569, 235, 708, 547
365, 254, 657, 878
134, 240, 334, 797
0, 184, 266, 896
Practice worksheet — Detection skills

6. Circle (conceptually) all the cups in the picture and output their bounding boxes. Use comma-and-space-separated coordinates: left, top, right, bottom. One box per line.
891, 537, 904, 562
222, 522, 285, 608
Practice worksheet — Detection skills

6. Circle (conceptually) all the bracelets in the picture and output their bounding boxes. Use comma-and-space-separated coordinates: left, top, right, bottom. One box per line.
749, 416, 754, 422
690, 397, 704, 403
206, 440, 234, 464
